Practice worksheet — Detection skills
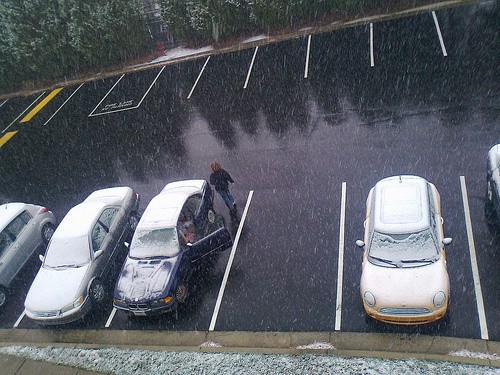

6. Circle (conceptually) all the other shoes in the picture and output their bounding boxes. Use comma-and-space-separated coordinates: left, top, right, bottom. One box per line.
230, 204, 237, 214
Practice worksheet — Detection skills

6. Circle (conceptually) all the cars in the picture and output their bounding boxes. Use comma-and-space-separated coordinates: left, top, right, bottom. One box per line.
24, 187, 141, 326
112, 178, 234, 318
0, 202, 58, 314
486, 143, 500, 227
354, 175, 454, 327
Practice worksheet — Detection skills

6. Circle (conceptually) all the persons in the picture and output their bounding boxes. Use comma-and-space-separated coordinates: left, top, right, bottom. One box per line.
156, 36, 167, 56
178, 223, 196, 242
210, 161, 239, 215
